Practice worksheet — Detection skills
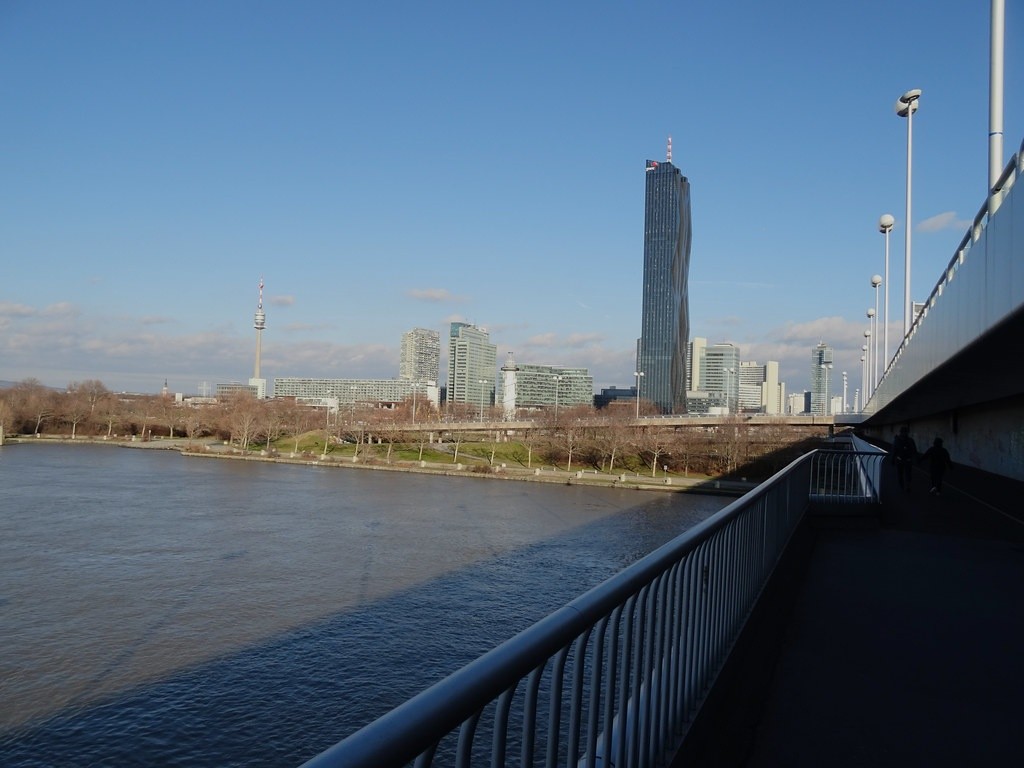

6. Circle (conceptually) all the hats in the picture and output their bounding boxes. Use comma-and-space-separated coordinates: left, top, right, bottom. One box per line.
899, 427, 910, 433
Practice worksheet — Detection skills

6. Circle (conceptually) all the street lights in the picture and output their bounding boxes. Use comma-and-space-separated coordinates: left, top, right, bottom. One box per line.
634, 372, 645, 418
723, 366, 735, 415
552, 375, 564, 419
896, 87, 921, 345
862, 345, 869, 409
409, 383, 421, 425
350, 386, 358, 425
821, 365, 834, 417
879, 213, 895, 378
479, 379, 488, 422
843, 371, 847, 413
866, 308, 876, 403
871, 274, 882, 392
860, 357, 865, 411
864, 329, 871, 406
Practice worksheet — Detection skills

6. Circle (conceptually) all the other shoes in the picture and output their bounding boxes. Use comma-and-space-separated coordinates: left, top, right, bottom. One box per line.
904, 484, 912, 496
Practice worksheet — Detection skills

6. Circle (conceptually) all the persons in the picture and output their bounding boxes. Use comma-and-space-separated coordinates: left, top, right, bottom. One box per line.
890, 427, 949, 500
